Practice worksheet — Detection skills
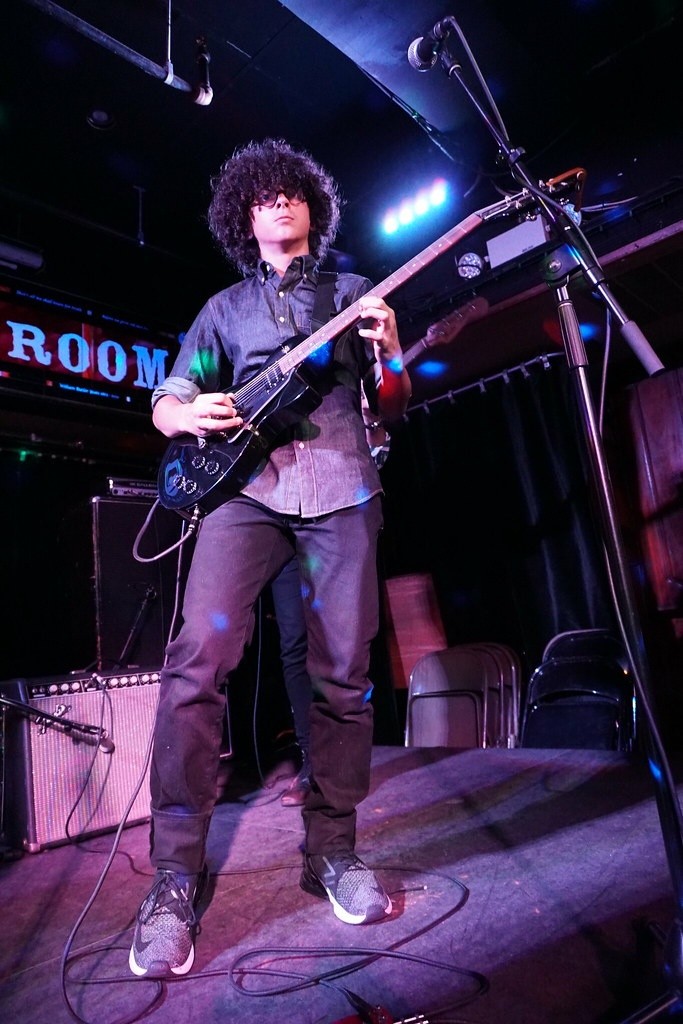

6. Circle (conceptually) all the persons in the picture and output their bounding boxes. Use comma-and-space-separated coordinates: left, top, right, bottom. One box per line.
126, 139, 409, 982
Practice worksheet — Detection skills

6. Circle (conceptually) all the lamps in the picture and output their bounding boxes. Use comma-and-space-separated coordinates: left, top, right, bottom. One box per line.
458, 204, 581, 279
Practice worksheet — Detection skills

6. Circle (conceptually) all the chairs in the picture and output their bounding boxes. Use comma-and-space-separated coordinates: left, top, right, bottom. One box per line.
522, 627, 642, 750
407, 644, 521, 749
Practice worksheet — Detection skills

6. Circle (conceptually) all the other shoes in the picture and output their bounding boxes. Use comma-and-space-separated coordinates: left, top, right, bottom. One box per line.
281, 778, 310, 807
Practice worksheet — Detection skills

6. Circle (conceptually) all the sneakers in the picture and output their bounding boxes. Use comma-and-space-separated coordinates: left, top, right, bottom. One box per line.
128, 873, 202, 979
301, 847, 392, 925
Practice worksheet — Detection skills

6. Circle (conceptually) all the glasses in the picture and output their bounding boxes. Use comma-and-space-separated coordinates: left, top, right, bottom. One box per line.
248, 184, 308, 208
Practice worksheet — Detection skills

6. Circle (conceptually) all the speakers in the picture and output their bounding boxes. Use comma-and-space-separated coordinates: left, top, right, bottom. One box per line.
0, 664, 162, 853
93, 497, 262, 801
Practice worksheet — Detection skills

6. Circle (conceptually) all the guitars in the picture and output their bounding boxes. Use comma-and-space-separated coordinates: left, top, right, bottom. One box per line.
153, 160, 596, 523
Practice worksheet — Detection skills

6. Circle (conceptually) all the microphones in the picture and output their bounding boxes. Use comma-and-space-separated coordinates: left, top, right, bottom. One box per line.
407, 15, 455, 72
98, 729, 113, 752
